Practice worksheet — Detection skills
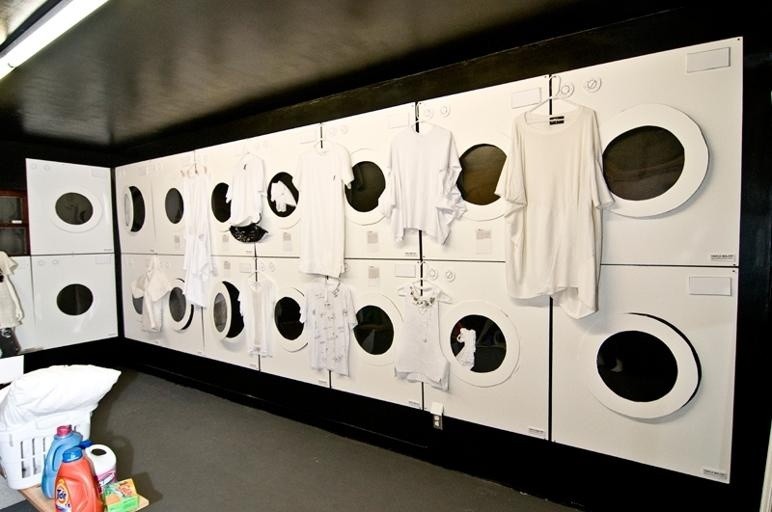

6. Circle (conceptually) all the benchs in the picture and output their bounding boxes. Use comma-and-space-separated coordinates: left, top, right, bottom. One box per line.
0, 461, 149, 512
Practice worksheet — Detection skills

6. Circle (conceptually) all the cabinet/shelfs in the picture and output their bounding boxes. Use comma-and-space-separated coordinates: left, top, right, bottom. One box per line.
1, 192, 31, 257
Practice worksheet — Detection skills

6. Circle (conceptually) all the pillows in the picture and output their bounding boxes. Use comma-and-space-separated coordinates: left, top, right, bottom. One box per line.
0, 365, 121, 427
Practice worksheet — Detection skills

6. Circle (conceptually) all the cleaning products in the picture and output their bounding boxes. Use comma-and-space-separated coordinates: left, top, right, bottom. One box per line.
42, 425, 84, 499
54, 448, 103, 511
550, 266, 739, 486
81, 437, 118, 494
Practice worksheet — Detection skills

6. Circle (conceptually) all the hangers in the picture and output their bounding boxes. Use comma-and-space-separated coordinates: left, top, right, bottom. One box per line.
247, 257, 265, 281
239, 142, 254, 163
396, 259, 454, 306
181, 153, 211, 177
311, 125, 330, 149
410, 103, 433, 131
524, 73, 582, 126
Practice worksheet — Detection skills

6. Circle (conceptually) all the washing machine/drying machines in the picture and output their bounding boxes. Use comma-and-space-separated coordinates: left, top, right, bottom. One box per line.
330, 258, 421, 409
26, 158, 116, 255
550, 37, 744, 266
255, 253, 328, 390
193, 138, 254, 255
322, 104, 422, 260
161, 256, 205, 357
120, 253, 160, 344
419, 74, 550, 261
114, 159, 154, 253
154, 151, 193, 255
32, 256, 119, 351
422, 261, 549, 442
207, 256, 259, 371
255, 123, 322, 255
